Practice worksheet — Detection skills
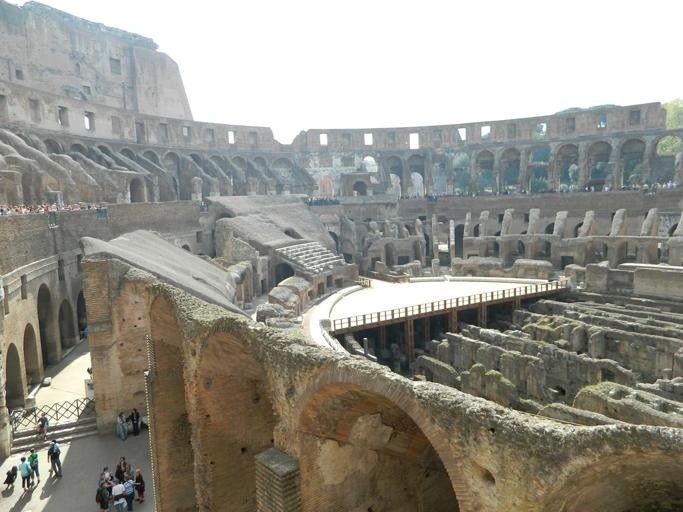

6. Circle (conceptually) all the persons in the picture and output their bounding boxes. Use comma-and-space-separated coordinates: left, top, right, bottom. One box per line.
26, 448, 40, 486
3, 466, 18, 490
0, 197, 98, 217
36, 411, 48, 434
35, 424, 45, 449
129, 408, 140, 436
15, 456, 32, 491
116, 411, 128, 440
95, 454, 146, 512
45, 439, 63, 478
